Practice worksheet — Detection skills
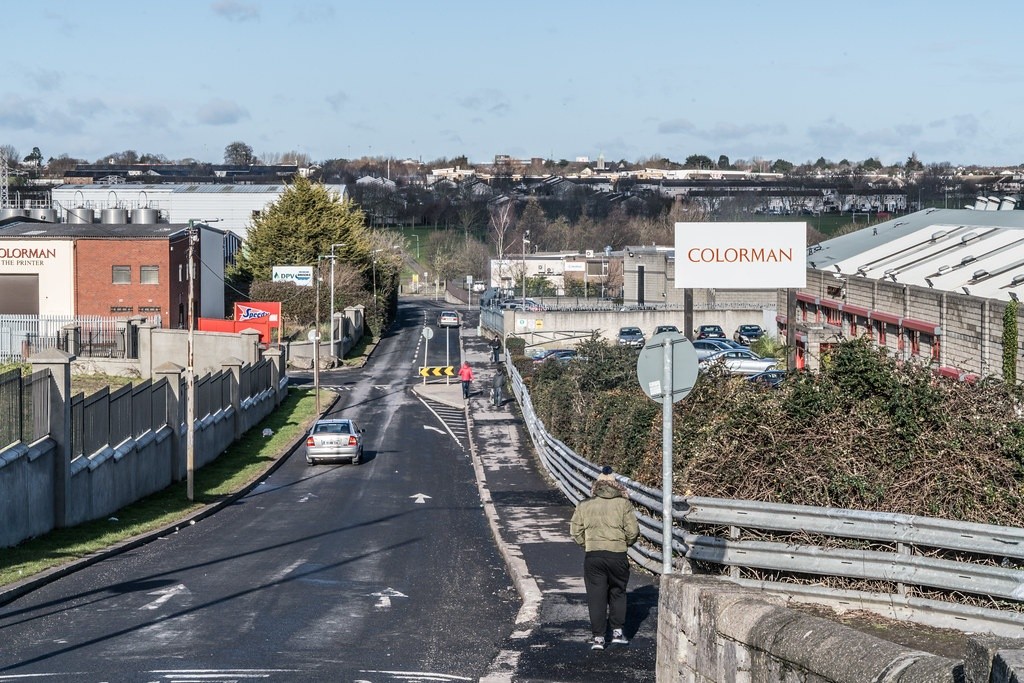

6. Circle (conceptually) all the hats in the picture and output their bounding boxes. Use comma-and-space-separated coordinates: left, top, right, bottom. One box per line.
597, 466, 615, 481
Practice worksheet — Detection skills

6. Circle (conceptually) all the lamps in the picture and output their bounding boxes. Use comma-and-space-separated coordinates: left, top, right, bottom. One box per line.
834, 265, 841, 273
859, 269, 867, 277
889, 274, 897, 282
1009, 292, 1019, 303
925, 279, 934, 288
809, 261, 816, 268
962, 287, 971, 295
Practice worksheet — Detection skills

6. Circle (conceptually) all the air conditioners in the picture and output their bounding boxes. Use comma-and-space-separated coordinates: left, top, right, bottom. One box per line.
537, 264, 546, 274
546, 267, 554, 274
586, 250, 594, 258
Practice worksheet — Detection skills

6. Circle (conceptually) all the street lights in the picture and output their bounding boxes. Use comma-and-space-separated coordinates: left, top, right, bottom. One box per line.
331, 244, 349, 357
397, 224, 403, 245
412, 235, 419, 258
315, 255, 339, 414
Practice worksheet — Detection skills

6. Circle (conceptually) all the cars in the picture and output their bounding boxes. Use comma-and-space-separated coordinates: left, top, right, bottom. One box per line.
698, 349, 785, 377
652, 325, 682, 336
692, 339, 733, 360
615, 327, 646, 349
436, 310, 459, 327
549, 355, 586, 369
305, 419, 365, 465
694, 324, 726, 340
733, 324, 767, 345
705, 337, 750, 351
746, 370, 787, 387
534, 348, 577, 362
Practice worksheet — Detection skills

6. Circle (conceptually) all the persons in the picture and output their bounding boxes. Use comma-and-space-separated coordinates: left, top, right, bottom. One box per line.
570, 466, 640, 649
491, 368, 506, 408
487, 335, 501, 365
459, 361, 473, 398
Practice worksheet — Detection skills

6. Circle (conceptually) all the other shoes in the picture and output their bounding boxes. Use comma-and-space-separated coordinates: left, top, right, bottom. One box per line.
591, 637, 605, 650
612, 630, 628, 644
463, 394, 466, 398
467, 395, 469, 398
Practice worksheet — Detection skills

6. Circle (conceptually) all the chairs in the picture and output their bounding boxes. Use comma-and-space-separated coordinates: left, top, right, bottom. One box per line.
340, 426, 348, 432
320, 426, 327, 432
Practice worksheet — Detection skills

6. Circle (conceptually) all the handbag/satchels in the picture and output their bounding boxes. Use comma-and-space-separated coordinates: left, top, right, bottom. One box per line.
489, 388, 495, 404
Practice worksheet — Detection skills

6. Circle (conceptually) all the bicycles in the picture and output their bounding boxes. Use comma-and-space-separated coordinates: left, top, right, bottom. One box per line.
487, 346, 498, 366
